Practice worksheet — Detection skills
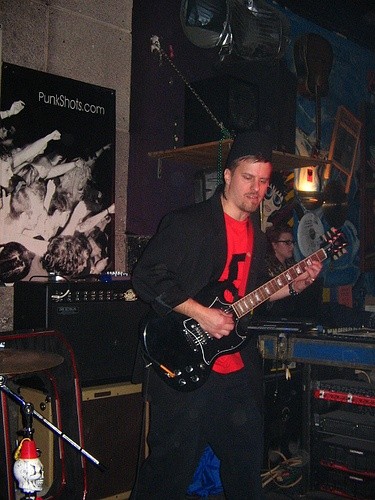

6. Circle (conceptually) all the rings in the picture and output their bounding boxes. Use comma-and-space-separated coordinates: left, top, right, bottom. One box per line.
312, 277, 315, 281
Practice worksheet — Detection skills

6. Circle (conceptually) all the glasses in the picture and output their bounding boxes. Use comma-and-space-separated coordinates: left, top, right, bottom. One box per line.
275, 240, 297, 245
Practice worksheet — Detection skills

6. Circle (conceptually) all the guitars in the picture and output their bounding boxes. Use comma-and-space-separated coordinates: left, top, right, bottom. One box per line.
141, 227, 350, 393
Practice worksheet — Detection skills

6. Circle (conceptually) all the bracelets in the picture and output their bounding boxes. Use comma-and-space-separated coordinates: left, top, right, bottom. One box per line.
107, 208, 109, 214
288, 283, 299, 297
101, 148, 104, 151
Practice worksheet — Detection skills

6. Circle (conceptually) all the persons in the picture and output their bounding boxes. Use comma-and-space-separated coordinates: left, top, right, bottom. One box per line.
263, 223, 300, 318
128, 131, 323, 500
0, 100, 116, 282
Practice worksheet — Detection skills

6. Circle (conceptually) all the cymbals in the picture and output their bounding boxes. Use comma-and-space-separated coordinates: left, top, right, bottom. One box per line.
0, 347, 65, 375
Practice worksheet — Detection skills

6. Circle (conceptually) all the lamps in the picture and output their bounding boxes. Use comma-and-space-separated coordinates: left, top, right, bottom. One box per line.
293, 165, 325, 211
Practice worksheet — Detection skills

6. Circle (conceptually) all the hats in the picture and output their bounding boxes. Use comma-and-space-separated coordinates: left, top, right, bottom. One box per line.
227, 132, 272, 162
